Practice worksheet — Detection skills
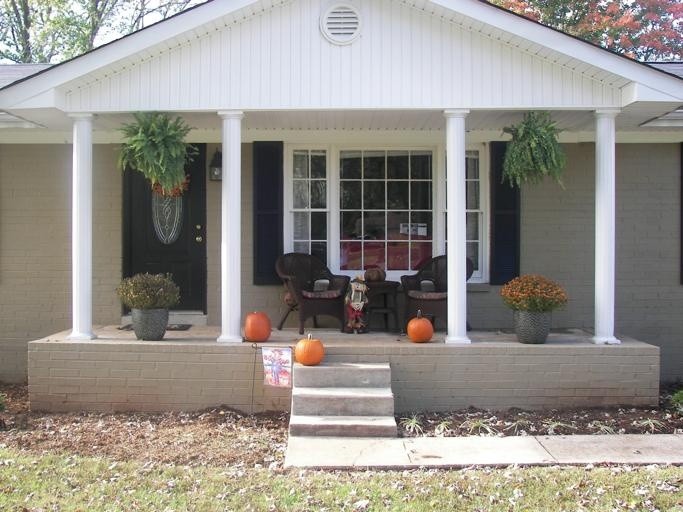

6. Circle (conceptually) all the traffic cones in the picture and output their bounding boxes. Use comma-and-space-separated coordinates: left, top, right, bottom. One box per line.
242, 310, 272, 344
404, 310, 434, 344
294, 333, 325, 367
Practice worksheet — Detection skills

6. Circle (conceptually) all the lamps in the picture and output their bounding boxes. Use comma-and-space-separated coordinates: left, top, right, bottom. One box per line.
274, 251, 350, 335
400, 253, 475, 335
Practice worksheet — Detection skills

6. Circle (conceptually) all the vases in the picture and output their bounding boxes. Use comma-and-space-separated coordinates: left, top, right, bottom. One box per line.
498, 272, 569, 314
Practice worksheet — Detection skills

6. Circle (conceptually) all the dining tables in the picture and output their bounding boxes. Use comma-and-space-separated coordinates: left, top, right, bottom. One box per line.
113, 270, 182, 340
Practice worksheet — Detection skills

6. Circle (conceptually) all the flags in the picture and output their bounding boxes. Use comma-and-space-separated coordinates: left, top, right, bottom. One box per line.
512, 307, 552, 344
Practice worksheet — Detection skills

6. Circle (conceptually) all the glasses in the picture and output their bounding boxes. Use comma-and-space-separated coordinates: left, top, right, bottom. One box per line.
208, 150, 222, 181
